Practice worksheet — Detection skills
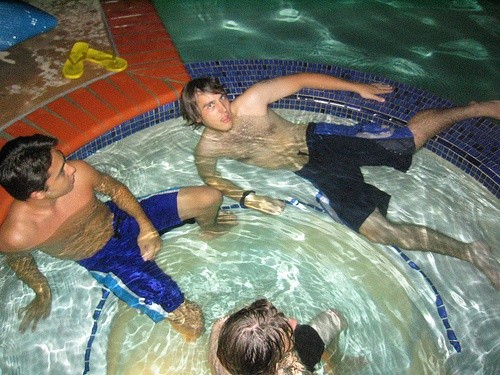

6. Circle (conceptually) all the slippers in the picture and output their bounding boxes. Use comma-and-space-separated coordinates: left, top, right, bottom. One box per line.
86, 48, 128, 72
63, 42, 89, 79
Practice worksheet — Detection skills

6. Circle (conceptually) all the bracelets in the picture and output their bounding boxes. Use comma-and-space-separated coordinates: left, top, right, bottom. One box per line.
239, 190, 255, 209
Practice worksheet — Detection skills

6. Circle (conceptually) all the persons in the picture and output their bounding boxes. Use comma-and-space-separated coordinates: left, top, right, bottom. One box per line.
209, 299, 348, 374
180, 72, 499, 290
0, 134, 237, 341
